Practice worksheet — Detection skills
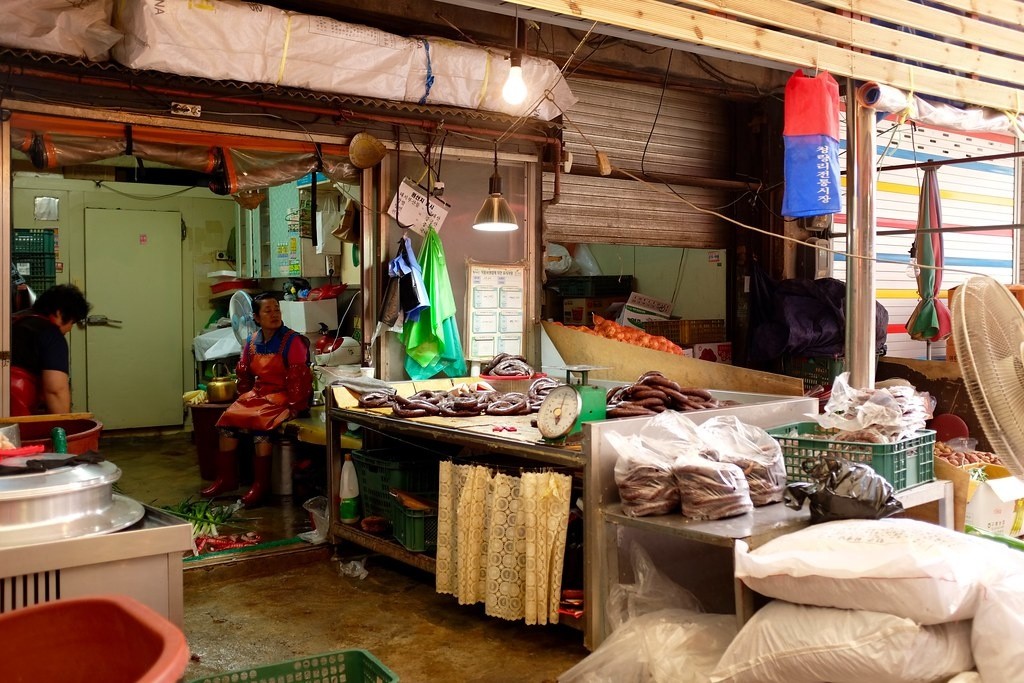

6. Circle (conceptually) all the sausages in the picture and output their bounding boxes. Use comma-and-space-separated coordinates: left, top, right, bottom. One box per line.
353, 353, 929, 519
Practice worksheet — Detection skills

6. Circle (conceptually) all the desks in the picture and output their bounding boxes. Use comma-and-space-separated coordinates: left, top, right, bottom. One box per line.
601, 480, 956, 632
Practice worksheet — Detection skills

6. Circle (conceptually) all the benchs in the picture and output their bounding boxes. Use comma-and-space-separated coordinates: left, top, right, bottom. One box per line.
275, 417, 362, 502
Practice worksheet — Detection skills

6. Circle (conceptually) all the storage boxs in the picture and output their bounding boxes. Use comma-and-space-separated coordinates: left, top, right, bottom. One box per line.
559, 275, 634, 299
615, 292, 675, 330
204, 304, 229, 329
957, 462, 1024, 538
350, 447, 430, 521
763, 421, 937, 494
10, 228, 57, 299
945, 284, 1024, 363
642, 318, 725, 349
388, 490, 438, 554
785, 349, 885, 390
563, 296, 629, 326
187, 648, 400, 683
279, 298, 338, 333
693, 342, 732, 365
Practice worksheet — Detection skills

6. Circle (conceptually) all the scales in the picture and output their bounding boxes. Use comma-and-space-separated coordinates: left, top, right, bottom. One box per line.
537, 364, 616, 449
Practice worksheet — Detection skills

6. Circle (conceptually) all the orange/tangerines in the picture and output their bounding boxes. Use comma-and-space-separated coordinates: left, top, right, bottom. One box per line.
549, 314, 686, 357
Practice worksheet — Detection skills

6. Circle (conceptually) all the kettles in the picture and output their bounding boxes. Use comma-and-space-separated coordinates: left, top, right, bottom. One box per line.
208, 361, 238, 403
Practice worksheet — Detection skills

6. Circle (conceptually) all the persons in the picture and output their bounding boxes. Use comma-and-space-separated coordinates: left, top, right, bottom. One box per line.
10, 283, 90, 416
202, 294, 312, 503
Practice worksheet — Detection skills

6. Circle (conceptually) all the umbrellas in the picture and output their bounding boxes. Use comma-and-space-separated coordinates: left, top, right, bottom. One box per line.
905, 159, 951, 360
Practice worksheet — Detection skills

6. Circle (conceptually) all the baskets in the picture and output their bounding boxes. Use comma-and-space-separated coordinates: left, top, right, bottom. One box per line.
764, 420, 937, 493
188, 648, 399, 683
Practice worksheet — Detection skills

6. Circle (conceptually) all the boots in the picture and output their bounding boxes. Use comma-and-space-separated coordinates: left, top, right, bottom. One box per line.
200, 449, 238, 498
242, 454, 272, 505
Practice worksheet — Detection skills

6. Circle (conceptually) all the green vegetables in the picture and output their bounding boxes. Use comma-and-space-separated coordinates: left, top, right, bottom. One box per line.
968, 465, 990, 483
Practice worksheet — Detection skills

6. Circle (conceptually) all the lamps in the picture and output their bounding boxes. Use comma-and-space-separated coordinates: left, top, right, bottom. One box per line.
472, 143, 519, 232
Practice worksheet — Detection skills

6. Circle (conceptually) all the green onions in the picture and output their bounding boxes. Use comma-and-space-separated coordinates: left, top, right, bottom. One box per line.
149, 496, 261, 537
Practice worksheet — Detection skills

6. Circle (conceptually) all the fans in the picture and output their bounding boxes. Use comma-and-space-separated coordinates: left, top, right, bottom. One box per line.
950, 276, 1024, 483
229, 290, 261, 346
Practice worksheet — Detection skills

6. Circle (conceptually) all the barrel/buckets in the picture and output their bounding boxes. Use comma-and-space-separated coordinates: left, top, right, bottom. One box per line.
192, 403, 251, 481
1, 592, 192, 683
17, 419, 104, 456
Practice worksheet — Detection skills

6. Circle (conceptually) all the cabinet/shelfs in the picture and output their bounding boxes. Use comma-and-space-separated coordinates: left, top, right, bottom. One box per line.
253, 180, 325, 278
233, 201, 253, 278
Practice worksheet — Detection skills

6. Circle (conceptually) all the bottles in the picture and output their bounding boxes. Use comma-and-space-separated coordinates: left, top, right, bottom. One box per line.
338, 454, 361, 525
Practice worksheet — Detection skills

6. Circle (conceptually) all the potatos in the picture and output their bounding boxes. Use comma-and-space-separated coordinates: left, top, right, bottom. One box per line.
934, 440, 1008, 467
